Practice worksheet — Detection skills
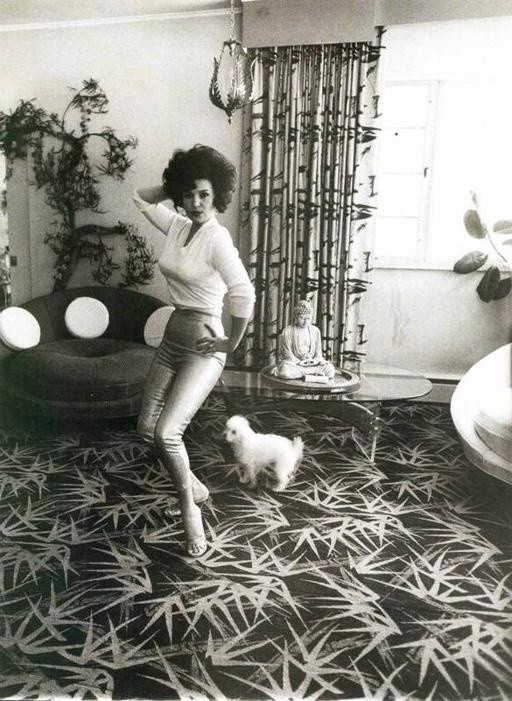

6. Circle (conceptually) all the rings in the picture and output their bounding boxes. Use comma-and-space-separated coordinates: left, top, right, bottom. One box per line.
212, 346, 216, 351
209, 342, 214, 347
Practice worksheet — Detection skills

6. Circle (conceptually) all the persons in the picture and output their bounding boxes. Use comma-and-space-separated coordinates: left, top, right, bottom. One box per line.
127, 142, 259, 563
269, 298, 338, 380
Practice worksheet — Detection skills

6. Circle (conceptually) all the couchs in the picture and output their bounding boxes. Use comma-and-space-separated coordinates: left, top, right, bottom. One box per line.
1, 286, 177, 432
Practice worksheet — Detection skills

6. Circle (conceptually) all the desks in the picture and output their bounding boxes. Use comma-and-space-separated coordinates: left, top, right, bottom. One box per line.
209, 356, 432, 464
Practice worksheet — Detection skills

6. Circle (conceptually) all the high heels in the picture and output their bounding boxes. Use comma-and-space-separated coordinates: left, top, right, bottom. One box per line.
182, 506, 207, 557
164, 487, 210, 519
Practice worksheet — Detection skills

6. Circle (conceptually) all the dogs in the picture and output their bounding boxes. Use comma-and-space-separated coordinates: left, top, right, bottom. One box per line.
220, 415, 306, 492
